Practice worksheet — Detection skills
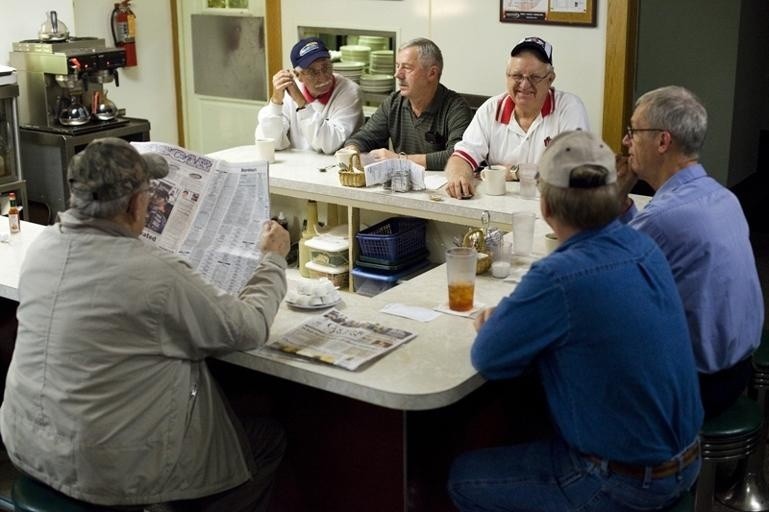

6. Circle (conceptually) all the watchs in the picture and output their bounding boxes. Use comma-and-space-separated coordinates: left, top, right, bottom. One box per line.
508, 163, 518, 181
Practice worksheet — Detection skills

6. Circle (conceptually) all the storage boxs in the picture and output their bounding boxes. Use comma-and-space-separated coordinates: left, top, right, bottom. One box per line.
304, 219, 369, 266
350, 261, 431, 299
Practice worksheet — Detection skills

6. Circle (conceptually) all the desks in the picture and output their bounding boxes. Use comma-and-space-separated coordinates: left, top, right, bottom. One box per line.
0, 217, 573, 511
202, 146, 654, 232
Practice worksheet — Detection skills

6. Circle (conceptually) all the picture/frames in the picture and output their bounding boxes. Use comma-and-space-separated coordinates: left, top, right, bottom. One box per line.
499, 0, 600, 30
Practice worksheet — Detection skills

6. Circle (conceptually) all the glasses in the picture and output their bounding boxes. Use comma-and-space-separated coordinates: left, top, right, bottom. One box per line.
301, 66, 329, 77
507, 72, 548, 83
628, 127, 664, 139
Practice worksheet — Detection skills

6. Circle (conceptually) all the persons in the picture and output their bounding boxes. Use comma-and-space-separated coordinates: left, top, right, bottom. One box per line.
340, 38, 471, 173
252, 35, 364, 165
444, 35, 590, 199
445, 125, 703, 510
0, 136, 293, 512
613, 83, 764, 416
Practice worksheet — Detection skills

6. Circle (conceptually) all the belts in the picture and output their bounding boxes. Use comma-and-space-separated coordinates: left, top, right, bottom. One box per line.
585, 437, 702, 481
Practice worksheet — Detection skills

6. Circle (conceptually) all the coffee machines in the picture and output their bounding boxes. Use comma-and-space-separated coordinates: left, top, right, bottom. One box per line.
8, 38, 129, 136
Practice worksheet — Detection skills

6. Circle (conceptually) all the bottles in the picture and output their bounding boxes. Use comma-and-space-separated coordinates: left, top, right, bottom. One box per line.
8, 193, 20, 234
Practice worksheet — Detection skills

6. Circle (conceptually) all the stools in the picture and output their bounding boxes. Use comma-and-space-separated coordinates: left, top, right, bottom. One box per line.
11, 472, 148, 512
732, 351, 767, 393
697, 393, 766, 511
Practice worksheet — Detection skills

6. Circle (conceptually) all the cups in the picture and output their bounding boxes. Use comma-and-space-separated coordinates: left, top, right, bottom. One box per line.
490, 242, 511, 279
519, 163, 538, 199
512, 212, 536, 258
445, 248, 478, 311
252, 139, 275, 165
334, 150, 357, 175
480, 165, 506, 196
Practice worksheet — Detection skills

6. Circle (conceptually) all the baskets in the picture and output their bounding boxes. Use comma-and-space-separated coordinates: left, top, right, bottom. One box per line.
356, 217, 426, 260
463, 229, 491, 274
338, 153, 365, 187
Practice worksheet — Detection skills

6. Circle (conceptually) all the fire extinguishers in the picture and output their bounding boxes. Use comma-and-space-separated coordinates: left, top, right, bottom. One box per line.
111, 0, 137, 67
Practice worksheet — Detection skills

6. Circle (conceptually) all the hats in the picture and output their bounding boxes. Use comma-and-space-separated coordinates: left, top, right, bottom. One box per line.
511, 36, 552, 64
290, 37, 330, 67
66, 137, 169, 202
534, 128, 617, 188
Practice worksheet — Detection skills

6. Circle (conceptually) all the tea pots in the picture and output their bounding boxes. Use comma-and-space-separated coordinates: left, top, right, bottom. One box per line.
38, 10, 69, 42
480, 211, 503, 263
453, 230, 492, 275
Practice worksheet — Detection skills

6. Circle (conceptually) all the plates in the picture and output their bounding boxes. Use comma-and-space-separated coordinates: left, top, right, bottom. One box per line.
287, 301, 343, 311
332, 35, 394, 94
